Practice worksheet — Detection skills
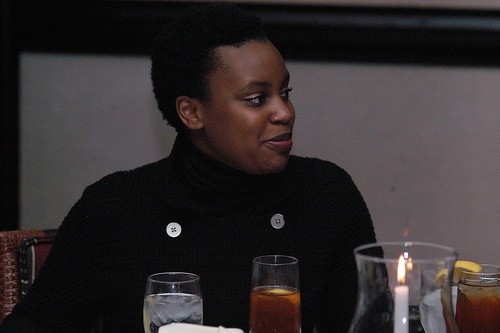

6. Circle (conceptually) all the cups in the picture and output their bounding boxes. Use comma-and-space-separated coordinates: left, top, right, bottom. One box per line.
251, 255, 301, 333
143, 273, 203, 333
420, 264, 500, 333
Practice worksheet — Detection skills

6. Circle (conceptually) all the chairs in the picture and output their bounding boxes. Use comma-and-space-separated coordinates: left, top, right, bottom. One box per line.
0, 229, 59, 325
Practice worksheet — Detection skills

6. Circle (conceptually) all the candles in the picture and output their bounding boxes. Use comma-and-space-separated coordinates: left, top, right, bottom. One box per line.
393, 254, 410, 333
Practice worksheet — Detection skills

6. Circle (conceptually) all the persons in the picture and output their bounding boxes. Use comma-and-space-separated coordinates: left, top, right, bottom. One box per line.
0, 3, 394, 333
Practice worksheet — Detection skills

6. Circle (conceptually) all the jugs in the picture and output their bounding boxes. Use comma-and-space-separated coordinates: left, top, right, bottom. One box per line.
350, 242, 460, 333
